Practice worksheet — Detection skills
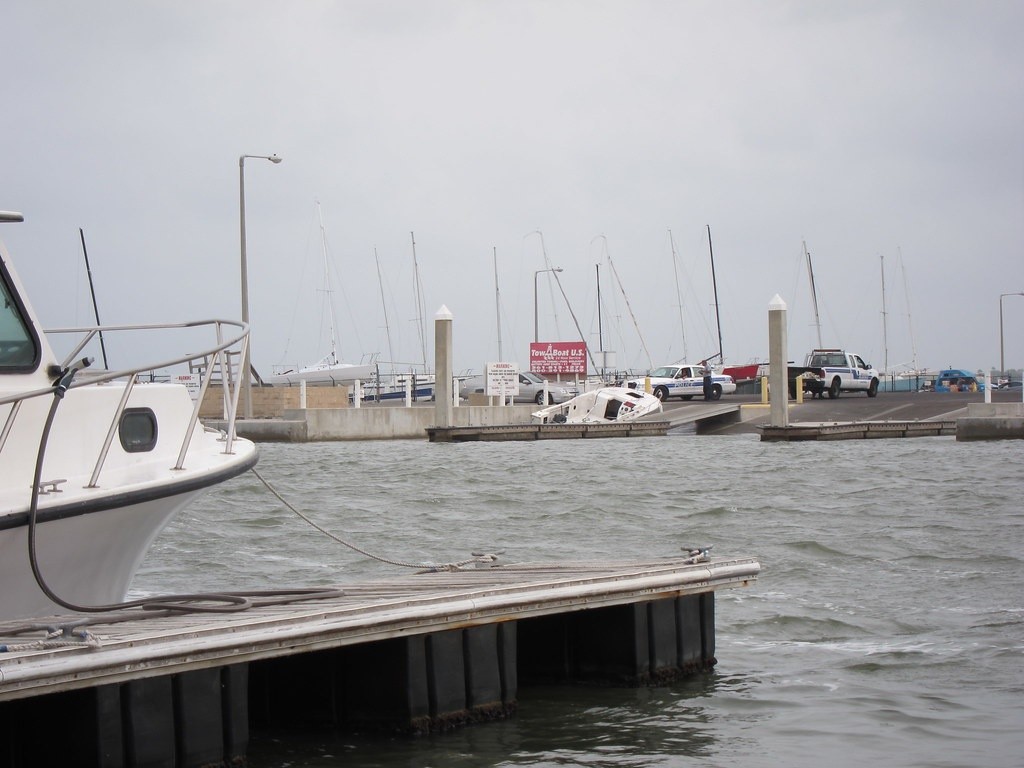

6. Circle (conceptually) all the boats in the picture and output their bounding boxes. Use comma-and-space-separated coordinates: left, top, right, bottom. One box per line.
532, 387, 663, 425
1, 212, 259, 621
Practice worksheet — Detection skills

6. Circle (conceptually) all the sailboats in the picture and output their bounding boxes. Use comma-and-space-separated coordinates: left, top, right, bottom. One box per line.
348, 247, 465, 404
876, 245, 933, 382
706, 224, 759, 387
271, 201, 377, 387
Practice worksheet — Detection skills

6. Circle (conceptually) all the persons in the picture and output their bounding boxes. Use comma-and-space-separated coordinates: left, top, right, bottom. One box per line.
968, 378, 978, 393
958, 377, 966, 392
699, 360, 712, 402
679, 369, 689, 380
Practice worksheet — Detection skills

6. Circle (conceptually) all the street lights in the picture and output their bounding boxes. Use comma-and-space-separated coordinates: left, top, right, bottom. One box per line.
240, 155, 282, 418
534, 268, 563, 343
1000, 293, 1024, 380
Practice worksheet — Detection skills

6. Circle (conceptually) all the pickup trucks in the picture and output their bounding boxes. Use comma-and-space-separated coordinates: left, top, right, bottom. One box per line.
788, 349, 880, 399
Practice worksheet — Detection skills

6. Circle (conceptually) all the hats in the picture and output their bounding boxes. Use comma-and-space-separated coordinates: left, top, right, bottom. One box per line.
701, 361, 707, 363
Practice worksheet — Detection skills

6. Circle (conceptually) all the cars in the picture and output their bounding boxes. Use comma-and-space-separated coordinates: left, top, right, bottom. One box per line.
998, 381, 1023, 391
627, 365, 737, 402
637, 398, 649, 406
504, 371, 580, 406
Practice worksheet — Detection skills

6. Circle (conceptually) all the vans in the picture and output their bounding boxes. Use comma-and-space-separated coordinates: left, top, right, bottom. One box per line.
934, 369, 985, 391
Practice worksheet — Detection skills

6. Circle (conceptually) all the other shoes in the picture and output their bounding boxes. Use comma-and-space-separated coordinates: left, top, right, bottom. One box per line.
704, 396, 708, 399
708, 398, 712, 402
